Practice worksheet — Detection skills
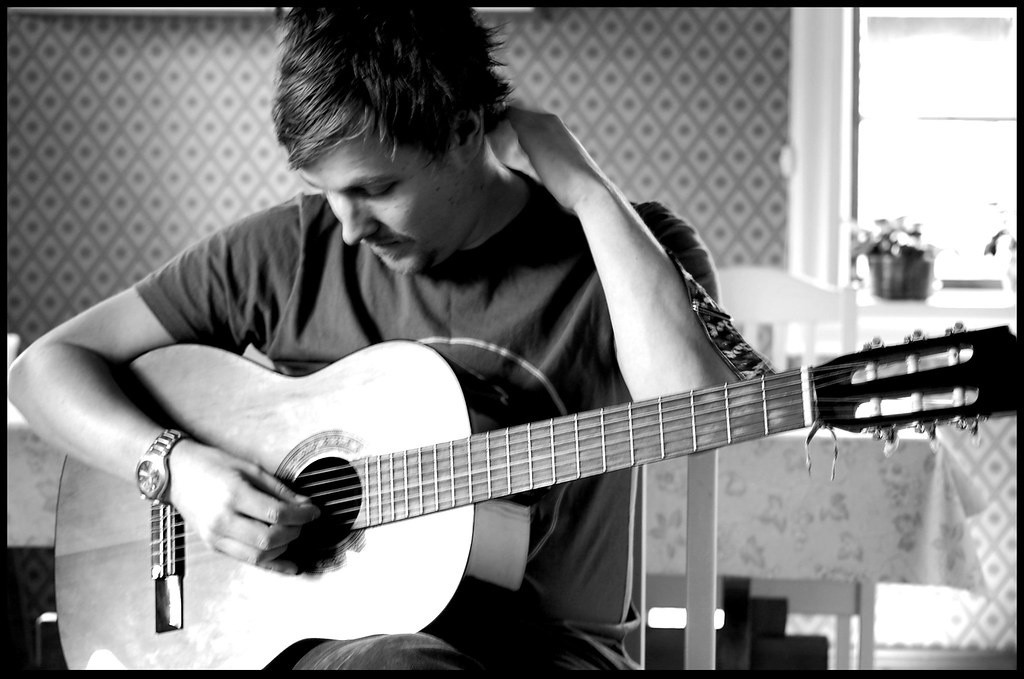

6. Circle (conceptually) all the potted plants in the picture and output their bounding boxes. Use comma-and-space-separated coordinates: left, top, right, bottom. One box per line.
851, 217, 942, 301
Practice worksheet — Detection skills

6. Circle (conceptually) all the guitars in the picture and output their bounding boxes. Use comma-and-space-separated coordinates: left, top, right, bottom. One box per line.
54, 322, 1024, 670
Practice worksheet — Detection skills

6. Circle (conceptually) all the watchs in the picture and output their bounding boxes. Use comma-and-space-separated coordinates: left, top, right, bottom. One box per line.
136, 428, 186, 504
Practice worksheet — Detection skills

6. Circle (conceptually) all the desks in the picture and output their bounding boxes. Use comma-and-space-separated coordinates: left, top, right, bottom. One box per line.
7, 397, 992, 670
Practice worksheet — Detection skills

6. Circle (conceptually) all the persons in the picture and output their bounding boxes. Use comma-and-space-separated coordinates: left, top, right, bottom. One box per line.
6, 6, 739, 670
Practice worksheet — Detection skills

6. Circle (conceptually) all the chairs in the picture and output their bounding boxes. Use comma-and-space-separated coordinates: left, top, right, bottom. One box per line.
645, 267, 858, 670
